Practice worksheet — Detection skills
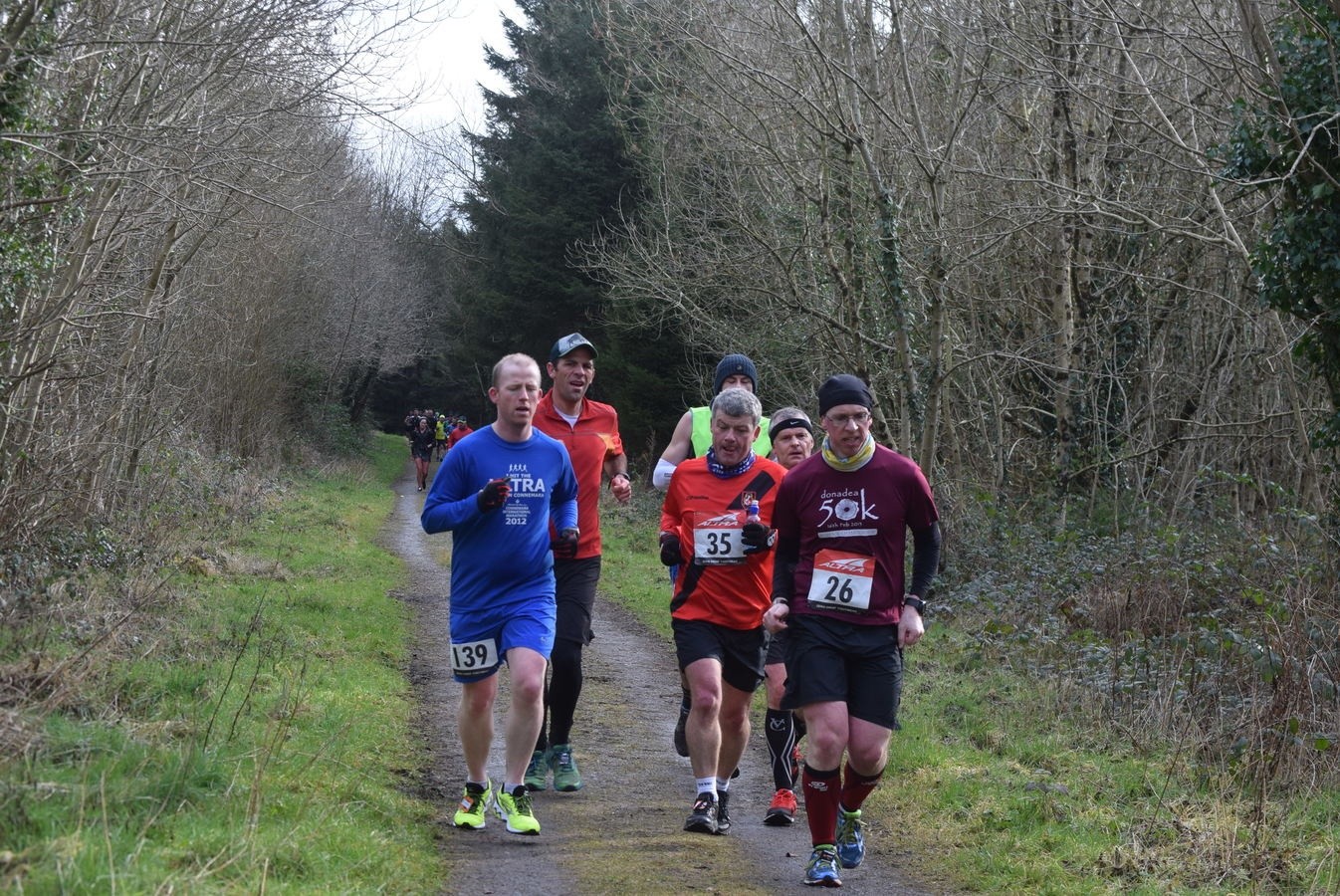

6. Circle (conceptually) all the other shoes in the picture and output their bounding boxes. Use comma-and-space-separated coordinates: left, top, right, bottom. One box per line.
422, 481, 425, 489
418, 486, 422, 492
437, 454, 443, 461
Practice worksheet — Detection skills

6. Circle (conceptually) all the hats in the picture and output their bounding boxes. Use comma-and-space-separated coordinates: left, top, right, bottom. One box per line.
439, 415, 446, 419
716, 354, 757, 394
817, 374, 874, 417
551, 333, 598, 360
457, 415, 467, 423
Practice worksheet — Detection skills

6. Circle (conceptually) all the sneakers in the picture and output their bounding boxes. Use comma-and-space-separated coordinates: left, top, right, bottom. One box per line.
545, 744, 582, 791
803, 845, 843, 885
835, 800, 866, 869
492, 783, 541, 836
684, 792, 718, 832
716, 789, 732, 835
453, 775, 495, 828
764, 788, 797, 823
522, 750, 548, 789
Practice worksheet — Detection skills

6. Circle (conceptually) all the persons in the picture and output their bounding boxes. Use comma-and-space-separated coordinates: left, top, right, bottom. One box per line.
403, 408, 476, 461
419, 353, 582, 835
522, 332, 631, 793
762, 375, 944, 887
760, 405, 816, 827
409, 414, 435, 492
652, 354, 771, 779
657, 387, 792, 832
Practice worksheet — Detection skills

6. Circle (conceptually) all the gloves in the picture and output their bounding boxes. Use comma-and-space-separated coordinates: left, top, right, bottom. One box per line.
548, 530, 578, 558
477, 477, 512, 510
659, 532, 687, 567
741, 524, 775, 555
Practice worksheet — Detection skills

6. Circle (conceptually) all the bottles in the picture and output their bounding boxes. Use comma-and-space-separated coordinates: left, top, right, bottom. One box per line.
744, 498, 763, 557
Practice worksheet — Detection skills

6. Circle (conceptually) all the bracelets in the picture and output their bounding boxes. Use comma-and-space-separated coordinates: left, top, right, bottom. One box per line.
773, 599, 788, 605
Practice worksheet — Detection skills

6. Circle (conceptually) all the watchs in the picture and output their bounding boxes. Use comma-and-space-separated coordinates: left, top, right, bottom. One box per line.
904, 597, 927, 615
607, 471, 631, 491
